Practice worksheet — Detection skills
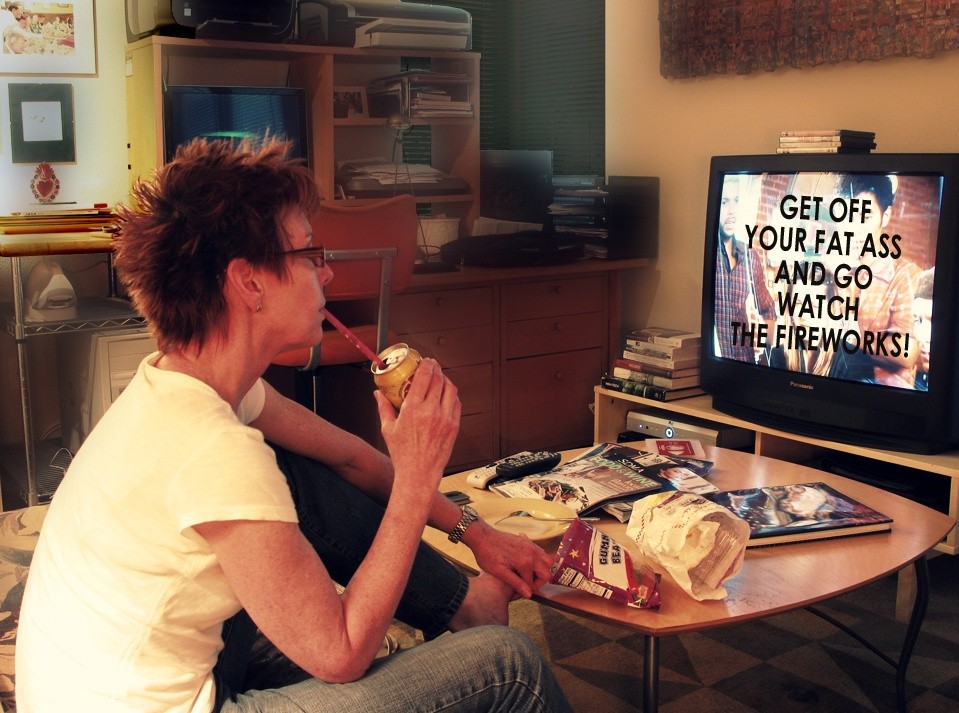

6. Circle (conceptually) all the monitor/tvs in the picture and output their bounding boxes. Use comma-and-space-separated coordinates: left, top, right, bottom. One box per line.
696, 152, 959, 453
164, 85, 310, 182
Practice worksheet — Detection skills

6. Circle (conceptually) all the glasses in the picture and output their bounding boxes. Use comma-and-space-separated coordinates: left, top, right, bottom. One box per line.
262, 245, 326, 271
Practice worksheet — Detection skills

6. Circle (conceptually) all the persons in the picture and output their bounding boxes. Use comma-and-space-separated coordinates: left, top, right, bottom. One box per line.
16, 126, 574, 713
714, 172, 918, 389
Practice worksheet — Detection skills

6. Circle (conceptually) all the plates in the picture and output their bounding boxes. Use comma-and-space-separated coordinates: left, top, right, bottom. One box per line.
467, 497, 578, 546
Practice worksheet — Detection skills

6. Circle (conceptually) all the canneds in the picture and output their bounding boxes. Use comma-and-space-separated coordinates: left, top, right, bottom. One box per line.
370, 343, 422, 416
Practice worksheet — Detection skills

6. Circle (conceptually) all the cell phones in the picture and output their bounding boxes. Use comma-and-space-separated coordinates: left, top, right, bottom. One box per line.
442, 490, 469, 506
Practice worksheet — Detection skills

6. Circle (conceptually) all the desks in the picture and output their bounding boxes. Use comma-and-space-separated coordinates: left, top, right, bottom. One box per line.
419, 435, 959, 713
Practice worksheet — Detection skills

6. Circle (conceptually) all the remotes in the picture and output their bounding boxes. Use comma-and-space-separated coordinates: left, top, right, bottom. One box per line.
497, 451, 562, 479
466, 451, 533, 490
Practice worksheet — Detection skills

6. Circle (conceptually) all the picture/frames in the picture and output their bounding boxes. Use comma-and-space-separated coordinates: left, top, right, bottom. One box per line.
0, 0, 99, 78
7, 80, 77, 167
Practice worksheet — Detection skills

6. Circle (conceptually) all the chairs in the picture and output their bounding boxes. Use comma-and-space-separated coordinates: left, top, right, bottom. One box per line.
270, 187, 423, 419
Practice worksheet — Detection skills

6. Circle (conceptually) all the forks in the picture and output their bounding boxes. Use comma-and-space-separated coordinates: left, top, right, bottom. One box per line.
494, 511, 600, 526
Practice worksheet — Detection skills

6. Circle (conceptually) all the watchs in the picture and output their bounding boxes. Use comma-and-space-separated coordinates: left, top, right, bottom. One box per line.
448, 505, 480, 544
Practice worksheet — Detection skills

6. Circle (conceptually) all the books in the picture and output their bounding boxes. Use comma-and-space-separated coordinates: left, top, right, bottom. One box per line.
411, 91, 452, 101
488, 441, 714, 523
700, 480, 894, 550
601, 326, 709, 404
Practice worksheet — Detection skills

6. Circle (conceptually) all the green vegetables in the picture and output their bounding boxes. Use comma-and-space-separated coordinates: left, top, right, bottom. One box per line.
529, 480, 576, 501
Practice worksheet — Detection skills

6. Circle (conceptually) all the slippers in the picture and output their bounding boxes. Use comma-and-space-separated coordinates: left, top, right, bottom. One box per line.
373, 634, 398, 659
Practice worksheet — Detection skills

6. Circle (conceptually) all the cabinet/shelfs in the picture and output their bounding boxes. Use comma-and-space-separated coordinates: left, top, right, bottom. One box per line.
585, 381, 959, 629
295, 253, 646, 476
123, 35, 484, 247
0, 234, 148, 507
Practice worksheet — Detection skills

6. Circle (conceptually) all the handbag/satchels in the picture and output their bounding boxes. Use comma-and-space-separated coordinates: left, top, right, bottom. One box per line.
440, 230, 585, 268
